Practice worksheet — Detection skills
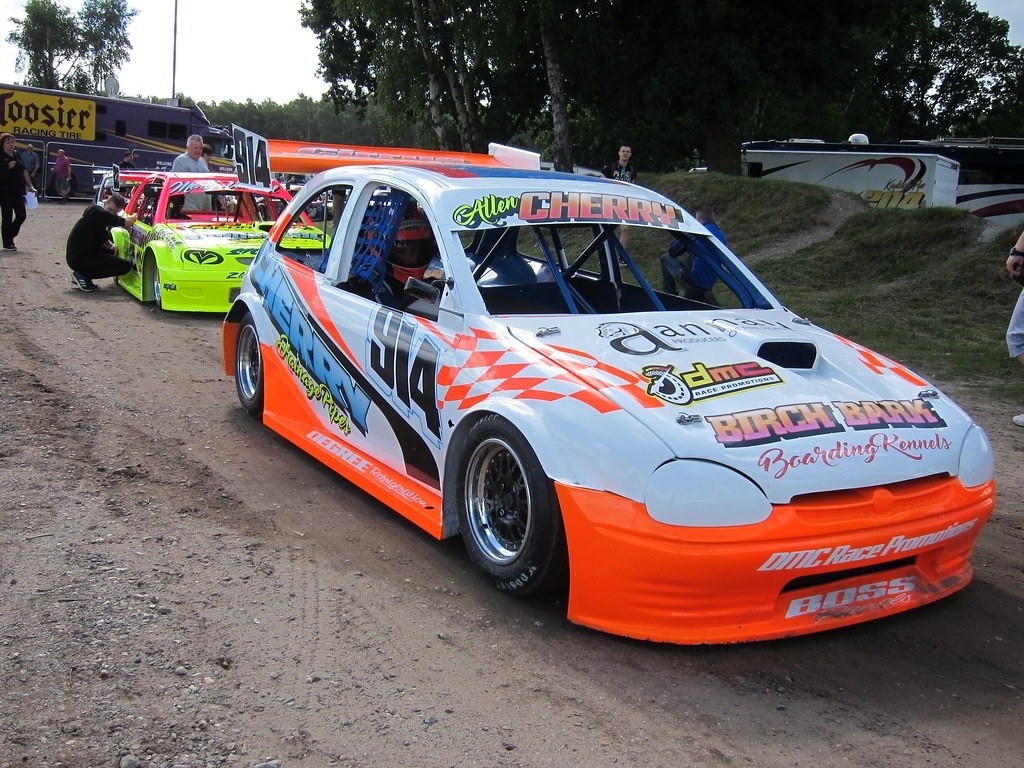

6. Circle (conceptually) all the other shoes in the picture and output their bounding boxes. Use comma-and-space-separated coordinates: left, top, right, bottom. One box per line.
618, 258, 627, 266
1012, 414, 1024, 427
63, 194, 69, 203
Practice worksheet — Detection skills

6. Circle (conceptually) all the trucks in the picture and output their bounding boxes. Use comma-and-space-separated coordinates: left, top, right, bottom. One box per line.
0, 83, 232, 198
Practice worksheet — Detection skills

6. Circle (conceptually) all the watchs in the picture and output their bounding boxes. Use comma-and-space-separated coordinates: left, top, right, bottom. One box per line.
1009, 247, 1024, 257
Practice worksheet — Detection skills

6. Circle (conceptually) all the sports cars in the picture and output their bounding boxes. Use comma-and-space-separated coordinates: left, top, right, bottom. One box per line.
92, 164, 392, 313
223, 136, 997, 645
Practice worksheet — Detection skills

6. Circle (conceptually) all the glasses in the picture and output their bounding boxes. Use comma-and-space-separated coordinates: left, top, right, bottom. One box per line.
129, 157, 134, 159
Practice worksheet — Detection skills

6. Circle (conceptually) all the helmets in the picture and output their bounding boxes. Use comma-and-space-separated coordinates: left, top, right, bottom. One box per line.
368, 202, 434, 286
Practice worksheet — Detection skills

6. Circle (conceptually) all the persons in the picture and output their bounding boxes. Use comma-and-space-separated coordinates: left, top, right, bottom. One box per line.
65, 193, 138, 292
0, 134, 38, 250
597, 143, 638, 268
118, 152, 137, 170
662, 207, 727, 307
153, 135, 227, 220
334, 200, 444, 314
1005, 230, 1024, 427
53, 149, 72, 204
21, 144, 41, 191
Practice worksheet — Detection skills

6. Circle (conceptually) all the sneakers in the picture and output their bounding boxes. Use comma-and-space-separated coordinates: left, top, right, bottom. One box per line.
2, 244, 17, 251
72, 280, 101, 290
71, 272, 94, 293
